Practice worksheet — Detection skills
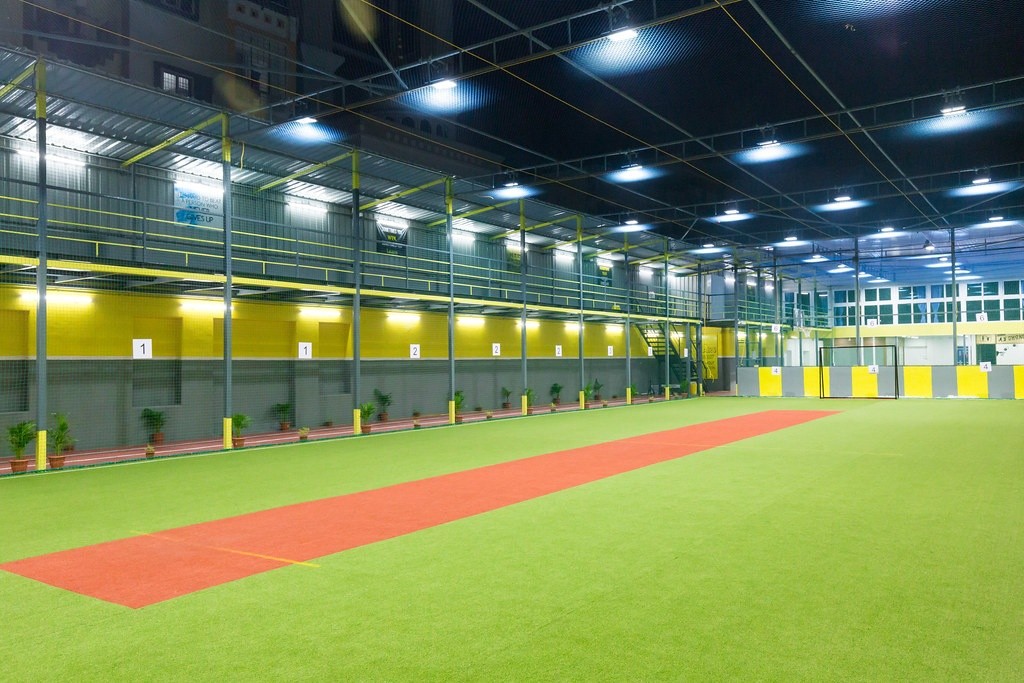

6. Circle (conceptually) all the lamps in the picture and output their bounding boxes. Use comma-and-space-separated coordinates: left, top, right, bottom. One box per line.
923, 239, 936, 251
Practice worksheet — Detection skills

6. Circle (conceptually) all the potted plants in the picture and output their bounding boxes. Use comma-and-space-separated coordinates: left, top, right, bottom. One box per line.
375, 388, 393, 421
299, 427, 310, 441
4, 419, 37, 472
47, 412, 72, 469
272, 402, 294, 430
141, 408, 170, 445
360, 402, 378, 433
145, 445, 155, 459
455, 378, 691, 431
413, 410, 422, 428
232, 413, 252, 447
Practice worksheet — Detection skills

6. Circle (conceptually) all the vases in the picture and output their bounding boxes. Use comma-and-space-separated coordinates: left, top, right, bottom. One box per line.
326, 422, 333, 427
